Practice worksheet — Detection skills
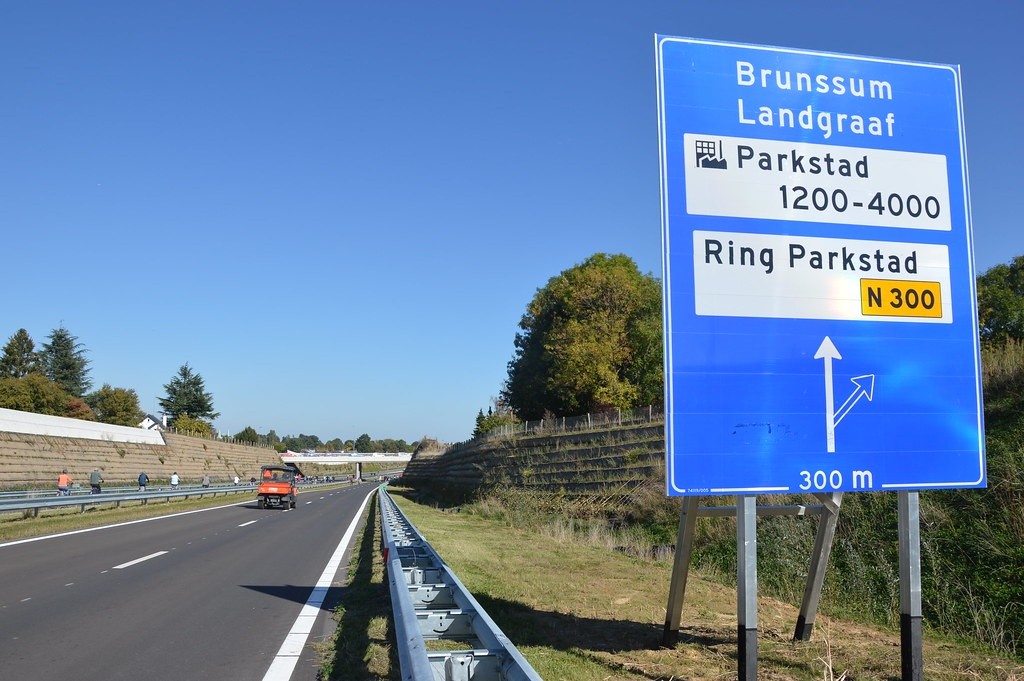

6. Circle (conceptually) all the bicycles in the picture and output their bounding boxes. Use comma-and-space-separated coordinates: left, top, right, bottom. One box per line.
138, 481, 148, 491
56, 483, 74, 497
170, 481, 179, 491
89, 481, 102, 495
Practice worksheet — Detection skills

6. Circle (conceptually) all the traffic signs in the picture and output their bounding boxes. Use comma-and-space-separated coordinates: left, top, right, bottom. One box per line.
654, 33, 988, 494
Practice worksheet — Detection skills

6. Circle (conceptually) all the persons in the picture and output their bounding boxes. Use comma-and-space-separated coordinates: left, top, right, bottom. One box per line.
234, 477, 240, 486
90, 468, 104, 494
170, 472, 180, 490
250, 476, 256, 485
296, 472, 362, 486
58, 470, 75, 496
138, 472, 150, 491
202, 474, 211, 488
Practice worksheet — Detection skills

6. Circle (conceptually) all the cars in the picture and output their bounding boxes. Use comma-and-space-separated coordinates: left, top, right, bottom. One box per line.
257, 464, 300, 510
